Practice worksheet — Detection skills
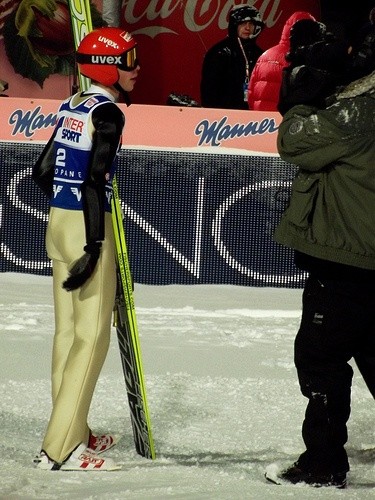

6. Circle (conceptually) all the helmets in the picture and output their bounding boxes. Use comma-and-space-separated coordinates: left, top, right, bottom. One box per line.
76, 27, 138, 83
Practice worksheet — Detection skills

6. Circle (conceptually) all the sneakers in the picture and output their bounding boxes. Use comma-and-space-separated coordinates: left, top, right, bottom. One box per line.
265, 462, 348, 489
88, 432, 122, 453
36, 442, 118, 471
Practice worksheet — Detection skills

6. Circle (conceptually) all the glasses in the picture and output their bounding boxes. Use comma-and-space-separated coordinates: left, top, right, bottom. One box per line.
77, 44, 138, 73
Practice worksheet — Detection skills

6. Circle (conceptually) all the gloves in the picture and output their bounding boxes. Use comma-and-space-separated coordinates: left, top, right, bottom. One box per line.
63, 243, 102, 290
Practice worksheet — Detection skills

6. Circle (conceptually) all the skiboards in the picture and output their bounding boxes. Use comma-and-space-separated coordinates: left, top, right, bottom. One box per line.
69, 0, 157, 461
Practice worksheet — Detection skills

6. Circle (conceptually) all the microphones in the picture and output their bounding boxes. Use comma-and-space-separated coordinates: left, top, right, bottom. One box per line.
249, 25, 262, 39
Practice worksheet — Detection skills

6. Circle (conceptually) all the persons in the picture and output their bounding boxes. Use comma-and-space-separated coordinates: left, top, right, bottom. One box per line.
31, 27, 141, 472
265, 10, 375, 488
248, 11, 326, 112
276, 19, 357, 116
200, 4, 266, 110
